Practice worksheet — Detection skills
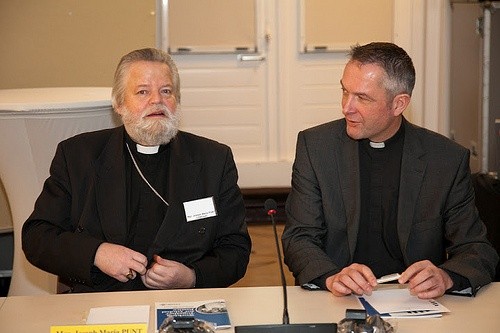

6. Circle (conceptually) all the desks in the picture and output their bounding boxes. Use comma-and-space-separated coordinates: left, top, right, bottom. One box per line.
0, 281, 500, 333
0, 86, 124, 297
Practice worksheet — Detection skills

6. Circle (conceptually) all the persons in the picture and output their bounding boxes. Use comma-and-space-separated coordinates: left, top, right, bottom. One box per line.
20, 49, 252, 293
281, 42, 500, 299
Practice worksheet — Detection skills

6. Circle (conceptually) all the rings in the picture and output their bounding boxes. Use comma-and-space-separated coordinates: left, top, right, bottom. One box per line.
126, 268, 134, 279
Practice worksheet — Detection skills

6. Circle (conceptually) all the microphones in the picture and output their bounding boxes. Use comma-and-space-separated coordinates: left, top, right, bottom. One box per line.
264, 199, 289, 325
